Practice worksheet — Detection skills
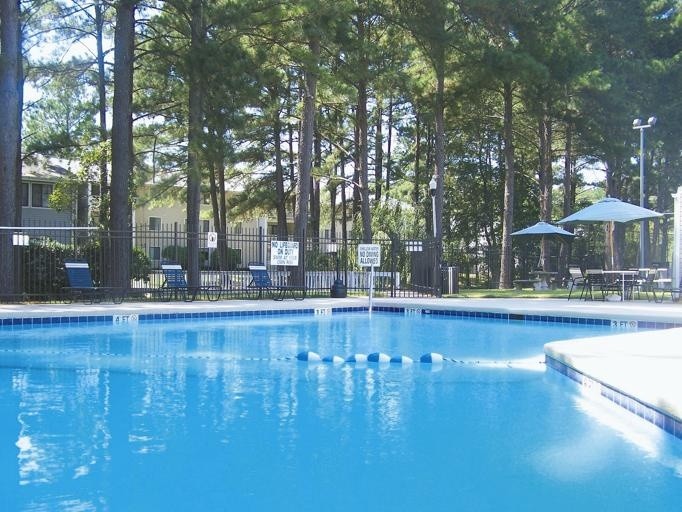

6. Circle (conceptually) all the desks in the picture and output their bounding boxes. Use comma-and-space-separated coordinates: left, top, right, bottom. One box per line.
529, 271, 559, 290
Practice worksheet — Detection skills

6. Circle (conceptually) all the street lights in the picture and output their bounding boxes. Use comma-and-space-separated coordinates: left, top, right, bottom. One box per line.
632, 116, 658, 274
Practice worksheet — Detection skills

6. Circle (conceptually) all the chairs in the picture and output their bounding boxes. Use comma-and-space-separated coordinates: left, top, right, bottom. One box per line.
60, 260, 306, 307
566, 266, 657, 305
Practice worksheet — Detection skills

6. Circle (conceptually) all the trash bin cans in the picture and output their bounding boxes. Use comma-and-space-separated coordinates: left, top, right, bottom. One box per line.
639, 269, 650, 279
658, 269, 668, 279
442, 266, 456, 294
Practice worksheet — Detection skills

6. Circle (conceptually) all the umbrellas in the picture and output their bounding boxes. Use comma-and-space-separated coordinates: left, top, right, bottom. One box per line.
508, 194, 666, 294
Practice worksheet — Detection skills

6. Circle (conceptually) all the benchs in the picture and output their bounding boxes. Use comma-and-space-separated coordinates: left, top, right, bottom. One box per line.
513, 279, 568, 291
